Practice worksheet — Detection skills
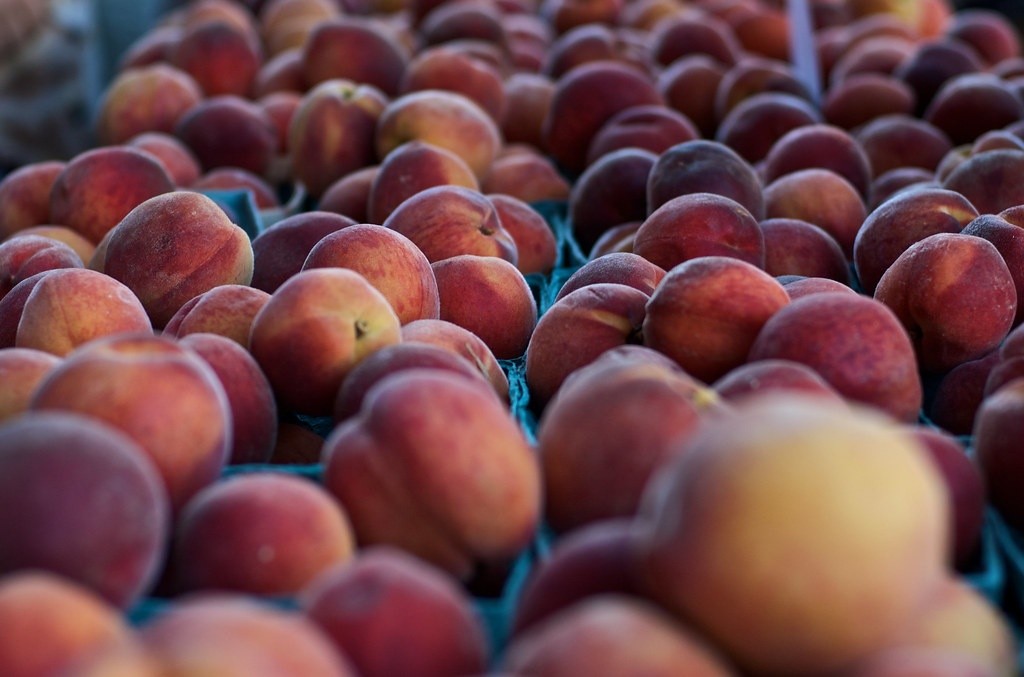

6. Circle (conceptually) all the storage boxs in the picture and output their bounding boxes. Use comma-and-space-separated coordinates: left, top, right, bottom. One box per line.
124, 199, 1024, 657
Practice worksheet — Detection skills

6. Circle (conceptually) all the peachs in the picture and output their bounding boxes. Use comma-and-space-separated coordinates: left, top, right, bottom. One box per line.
0, 0, 1024, 677
